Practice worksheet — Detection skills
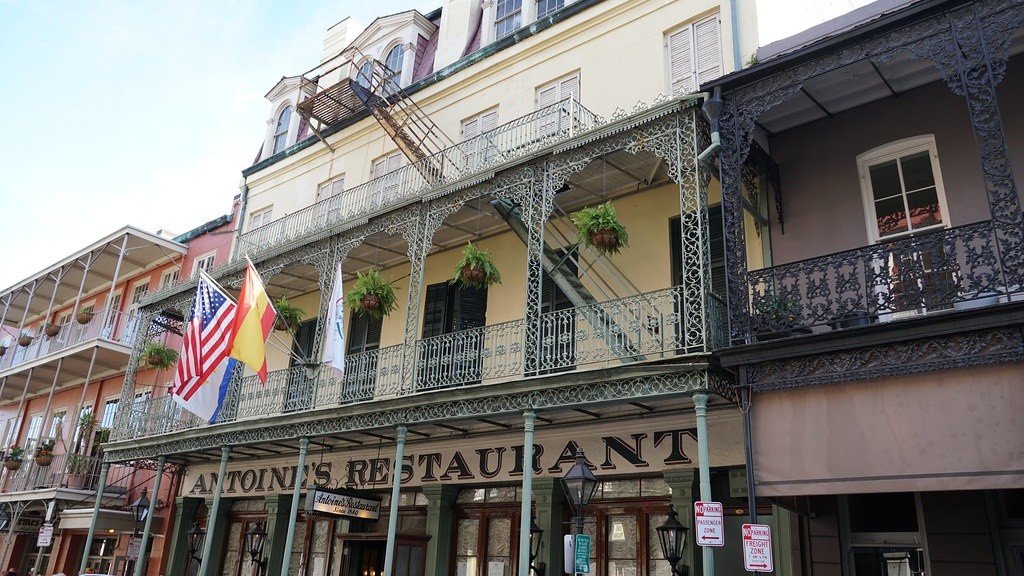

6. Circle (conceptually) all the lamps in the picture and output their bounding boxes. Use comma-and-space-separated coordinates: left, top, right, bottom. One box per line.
247, 520, 269, 576
185, 523, 206, 566
528, 512, 547, 575
656, 502, 692, 576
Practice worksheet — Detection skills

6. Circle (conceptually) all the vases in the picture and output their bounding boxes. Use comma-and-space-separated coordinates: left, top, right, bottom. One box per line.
34, 455, 55, 466
827, 310, 879, 331
951, 290, 998, 310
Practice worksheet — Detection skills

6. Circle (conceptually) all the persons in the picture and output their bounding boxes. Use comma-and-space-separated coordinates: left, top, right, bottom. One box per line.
2, 567, 94, 576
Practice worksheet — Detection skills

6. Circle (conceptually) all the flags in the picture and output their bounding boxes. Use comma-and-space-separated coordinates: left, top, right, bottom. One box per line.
323, 263, 344, 383
169, 272, 238, 425
225, 266, 277, 388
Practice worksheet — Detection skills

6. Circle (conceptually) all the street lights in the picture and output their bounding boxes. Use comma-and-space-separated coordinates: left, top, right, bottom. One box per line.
559, 447, 600, 576
125, 487, 151, 576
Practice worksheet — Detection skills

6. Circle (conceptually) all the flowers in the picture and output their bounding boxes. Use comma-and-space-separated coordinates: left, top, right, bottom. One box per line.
34, 441, 53, 457
2, 445, 24, 461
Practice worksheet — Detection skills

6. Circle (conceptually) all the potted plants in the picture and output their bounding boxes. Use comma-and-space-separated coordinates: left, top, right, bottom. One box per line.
345, 268, 400, 320
751, 297, 812, 343
450, 239, 502, 291
45, 323, 61, 335
77, 310, 94, 324
54, 409, 111, 491
569, 199, 631, 255
272, 296, 305, 332
18, 332, 33, 346
140, 340, 181, 370
